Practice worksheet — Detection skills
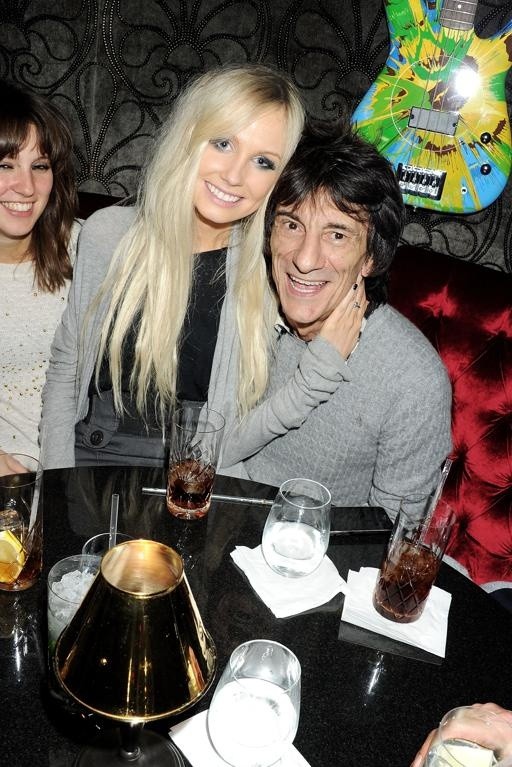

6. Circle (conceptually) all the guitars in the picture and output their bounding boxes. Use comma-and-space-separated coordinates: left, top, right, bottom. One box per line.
349, 0, 511, 214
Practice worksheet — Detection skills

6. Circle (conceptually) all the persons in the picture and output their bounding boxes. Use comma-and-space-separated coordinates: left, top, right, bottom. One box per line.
214, 117, 453, 540
2, 86, 84, 478
36, 59, 307, 472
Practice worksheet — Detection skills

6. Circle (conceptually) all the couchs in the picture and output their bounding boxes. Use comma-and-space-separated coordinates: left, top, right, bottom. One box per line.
75, 189, 511, 594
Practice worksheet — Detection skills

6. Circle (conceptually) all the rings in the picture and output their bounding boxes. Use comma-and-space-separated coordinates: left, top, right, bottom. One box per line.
353, 303, 360, 309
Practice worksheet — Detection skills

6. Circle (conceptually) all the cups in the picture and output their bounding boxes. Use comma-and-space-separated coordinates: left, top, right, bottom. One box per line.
0, 452, 45, 594
166, 405, 226, 522
80, 532, 138, 577
47, 553, 105, 718
373, 492, 458, 624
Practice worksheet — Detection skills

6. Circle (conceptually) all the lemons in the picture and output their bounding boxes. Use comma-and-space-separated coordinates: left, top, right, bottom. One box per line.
0, 529, 29, 584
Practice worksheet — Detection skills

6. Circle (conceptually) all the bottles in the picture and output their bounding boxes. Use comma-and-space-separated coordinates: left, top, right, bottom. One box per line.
262, 479, 334, 579
205, 638, 304, 767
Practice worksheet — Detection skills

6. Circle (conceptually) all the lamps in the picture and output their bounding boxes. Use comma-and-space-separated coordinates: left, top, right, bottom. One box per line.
50, 535, 220, 767
